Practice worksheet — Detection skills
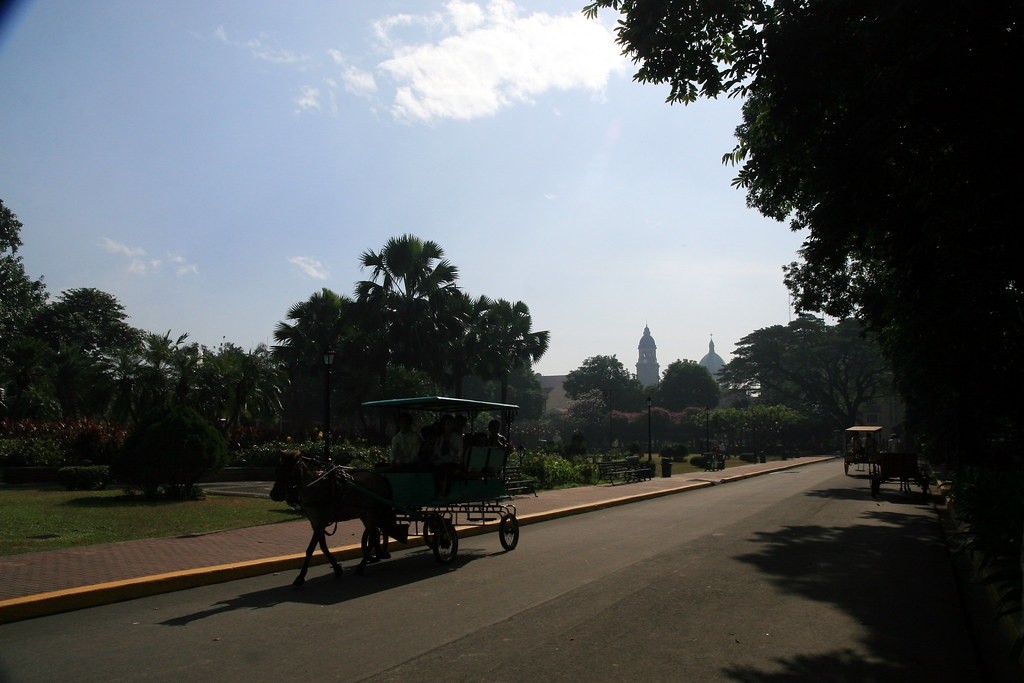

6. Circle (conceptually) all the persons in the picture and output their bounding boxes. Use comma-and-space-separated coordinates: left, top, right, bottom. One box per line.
851, 432, 864, 450
892, 435, 897, 448
388, 412, 421, 465
421, 414, 507, 464
865, 433, 878, 452
714, 441, 730, 460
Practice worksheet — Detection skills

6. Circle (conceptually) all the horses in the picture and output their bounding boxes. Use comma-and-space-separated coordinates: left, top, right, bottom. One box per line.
270, 447, 398, 589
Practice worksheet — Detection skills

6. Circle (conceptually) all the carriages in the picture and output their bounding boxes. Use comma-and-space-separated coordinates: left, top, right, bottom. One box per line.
269, 396, 521, 594
871, 447, 924, 500
842, 426, 886, 475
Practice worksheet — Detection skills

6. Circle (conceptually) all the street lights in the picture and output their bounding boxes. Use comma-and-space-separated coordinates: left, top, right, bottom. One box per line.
705, 405, 711, 453
646, 396, 653, 462
500, 366, 511, 436
321, 347, 336, 465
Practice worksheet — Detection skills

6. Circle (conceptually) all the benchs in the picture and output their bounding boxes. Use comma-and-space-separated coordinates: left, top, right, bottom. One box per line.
461, 447, 505, 480
594, 455, 651, 487
702, 452, 725, 472
505, 465, 538, 497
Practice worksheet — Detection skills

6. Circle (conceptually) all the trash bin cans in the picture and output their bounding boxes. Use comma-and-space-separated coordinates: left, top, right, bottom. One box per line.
660, 458, 673, 477
